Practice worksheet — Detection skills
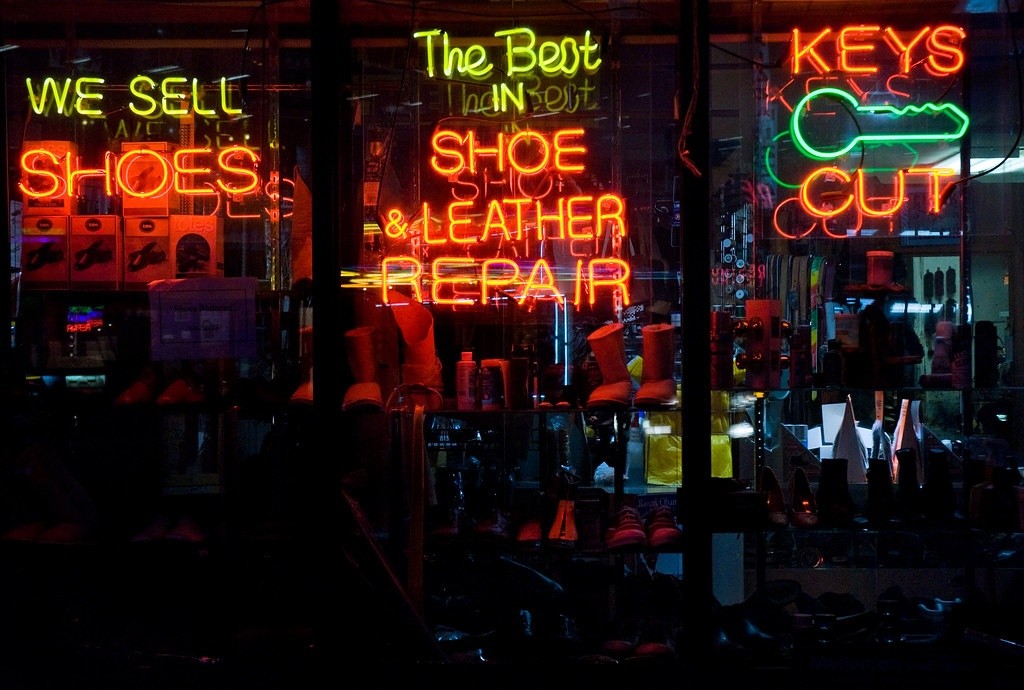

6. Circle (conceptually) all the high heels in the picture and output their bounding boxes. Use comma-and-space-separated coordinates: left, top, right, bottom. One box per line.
788, 466, 820, 527
758, 467, 788, 525
866, 458, 895, 523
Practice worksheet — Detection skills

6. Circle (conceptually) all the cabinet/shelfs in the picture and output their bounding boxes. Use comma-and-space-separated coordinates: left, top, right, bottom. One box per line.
423, 406, 664, 552
729, 388, 1024, 664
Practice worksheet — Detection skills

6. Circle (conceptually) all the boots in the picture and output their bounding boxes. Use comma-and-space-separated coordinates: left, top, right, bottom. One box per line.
895, 447, 924, 527
586, 321, 632, 409
918, 448, 961, 534
289, 327, 314, 405
632, 322, 676, 409
970, 465, 1024, 536
341, 327, 385, 410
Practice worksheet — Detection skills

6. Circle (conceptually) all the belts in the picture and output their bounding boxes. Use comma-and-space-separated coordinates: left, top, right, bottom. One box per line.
721, 254, 832, 491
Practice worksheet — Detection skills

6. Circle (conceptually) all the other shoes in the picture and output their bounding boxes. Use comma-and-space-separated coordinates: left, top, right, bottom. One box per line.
157, 377, 206, 405
134, 520, 206, 544
516, 519, 543, 542
543, 613, 582, 644
604, 505, 651, 548
539, 379, 583, 410
644, 507, 683, 546
602, 620, 680, 658
428, 505, 460, 538
547, 499, 580, 545
4, 521, 83, 544
493, 607, 533, 639
115, 378, 153, 406
468, 508, 511, 542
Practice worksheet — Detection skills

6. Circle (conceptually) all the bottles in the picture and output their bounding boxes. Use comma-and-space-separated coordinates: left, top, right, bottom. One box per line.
930, 336, 953, 392
457, 352, 478, 411
